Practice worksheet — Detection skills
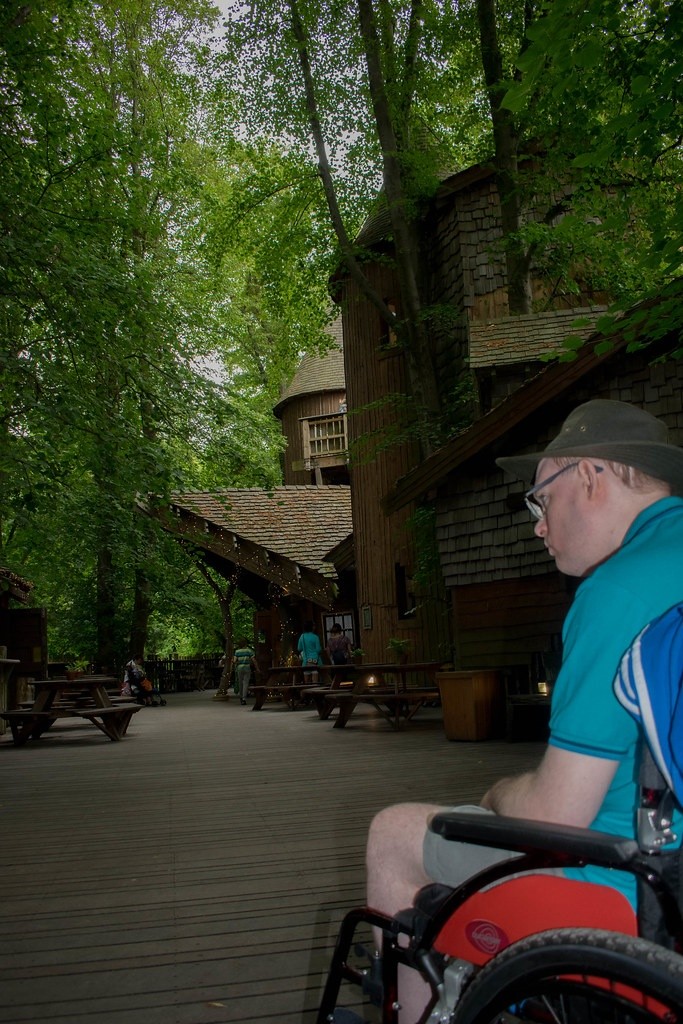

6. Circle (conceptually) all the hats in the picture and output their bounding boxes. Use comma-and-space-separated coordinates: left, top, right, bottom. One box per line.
496, 399, 683, 489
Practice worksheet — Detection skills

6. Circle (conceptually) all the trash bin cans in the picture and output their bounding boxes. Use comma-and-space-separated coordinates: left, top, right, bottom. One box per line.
434, 666, 497, 744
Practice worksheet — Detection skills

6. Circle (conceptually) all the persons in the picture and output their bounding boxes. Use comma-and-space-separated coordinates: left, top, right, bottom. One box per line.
124, 654, 153, 706
326, 623, 353, 682
234, 639, 260, 705
366, 399, 683, 1024
136, 671, 160, 704
297, 622, 322, 688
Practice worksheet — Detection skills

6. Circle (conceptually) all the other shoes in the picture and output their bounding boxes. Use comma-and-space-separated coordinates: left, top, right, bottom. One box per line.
146, 700, 157, 706
240, 697, 246, 705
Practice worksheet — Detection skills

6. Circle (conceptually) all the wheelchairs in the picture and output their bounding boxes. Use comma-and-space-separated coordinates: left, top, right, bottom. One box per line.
312, 606, 683, 1024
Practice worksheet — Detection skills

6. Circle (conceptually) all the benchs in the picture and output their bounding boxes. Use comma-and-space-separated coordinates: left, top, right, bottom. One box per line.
249, 682, 439, 729
0, 690, 145, 745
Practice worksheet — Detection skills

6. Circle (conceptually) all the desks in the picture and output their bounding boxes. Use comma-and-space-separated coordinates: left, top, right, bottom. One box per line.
435, 667, 510, 741
319, 663, 406, 719
333, 661, 455, 729
22, 677, 121, 741
251, 665, 352, 712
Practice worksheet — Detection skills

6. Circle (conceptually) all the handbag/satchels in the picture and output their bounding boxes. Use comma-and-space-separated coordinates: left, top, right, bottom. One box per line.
306, 657, 318, 666
140, 678, 152, 691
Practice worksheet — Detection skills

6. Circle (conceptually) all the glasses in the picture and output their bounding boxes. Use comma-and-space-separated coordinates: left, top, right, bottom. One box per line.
524, 461, 604, 520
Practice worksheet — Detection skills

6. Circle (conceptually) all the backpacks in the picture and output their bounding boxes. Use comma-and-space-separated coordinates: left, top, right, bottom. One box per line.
331, 636, 346, 665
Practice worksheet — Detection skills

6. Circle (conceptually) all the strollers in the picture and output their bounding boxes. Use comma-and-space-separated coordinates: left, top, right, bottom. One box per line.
127, 665, 166, 707
